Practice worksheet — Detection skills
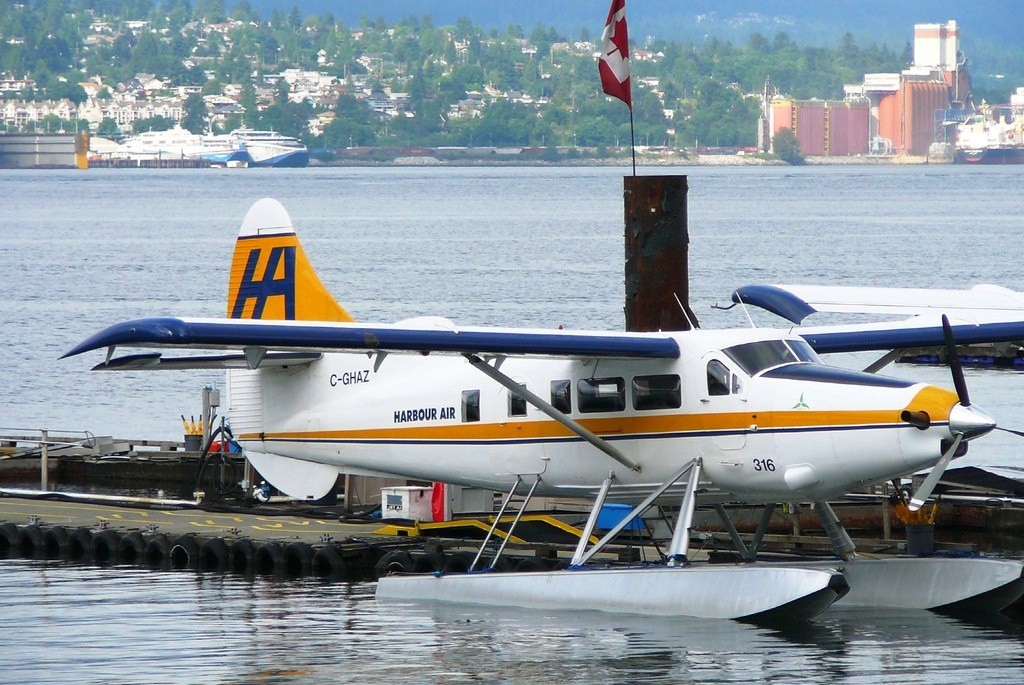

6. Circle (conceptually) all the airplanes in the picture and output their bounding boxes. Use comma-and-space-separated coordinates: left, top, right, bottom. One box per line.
57, 194, 1024, 574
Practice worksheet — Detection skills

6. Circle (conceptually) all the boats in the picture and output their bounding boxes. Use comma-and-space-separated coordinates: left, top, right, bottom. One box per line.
0, 427, 263, 489
74, 129, 309, 167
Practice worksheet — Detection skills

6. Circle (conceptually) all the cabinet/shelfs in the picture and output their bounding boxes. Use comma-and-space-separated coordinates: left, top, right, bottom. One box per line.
381, 486, 434, 521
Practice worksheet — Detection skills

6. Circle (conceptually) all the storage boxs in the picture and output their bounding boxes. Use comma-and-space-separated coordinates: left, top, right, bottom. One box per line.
210, 439, 244, 464
597, 503, 645, 530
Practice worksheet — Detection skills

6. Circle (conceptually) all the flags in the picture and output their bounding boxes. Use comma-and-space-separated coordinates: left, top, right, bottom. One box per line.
598, 0, 631, 108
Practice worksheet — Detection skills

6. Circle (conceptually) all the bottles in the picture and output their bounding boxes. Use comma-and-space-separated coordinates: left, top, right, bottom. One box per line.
259, 481, 269, 498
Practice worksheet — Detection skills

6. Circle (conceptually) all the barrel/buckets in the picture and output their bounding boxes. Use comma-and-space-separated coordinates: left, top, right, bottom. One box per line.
905, 523, 935, 553
184, 435, 202, 451
